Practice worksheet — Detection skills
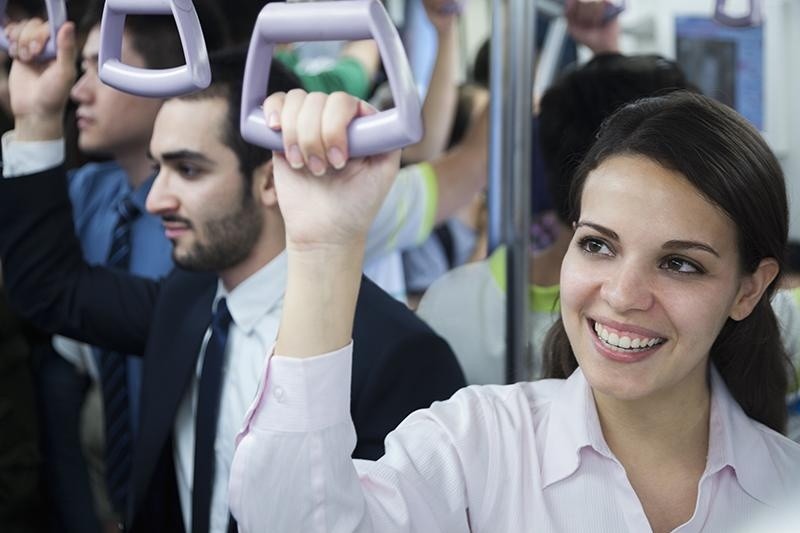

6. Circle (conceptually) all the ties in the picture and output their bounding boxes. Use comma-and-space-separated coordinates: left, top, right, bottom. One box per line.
190, 297, 232, 533
102, 200, 145, 505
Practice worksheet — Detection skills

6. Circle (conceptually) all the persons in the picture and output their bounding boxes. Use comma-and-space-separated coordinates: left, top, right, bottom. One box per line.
0, 0, 800, 533
